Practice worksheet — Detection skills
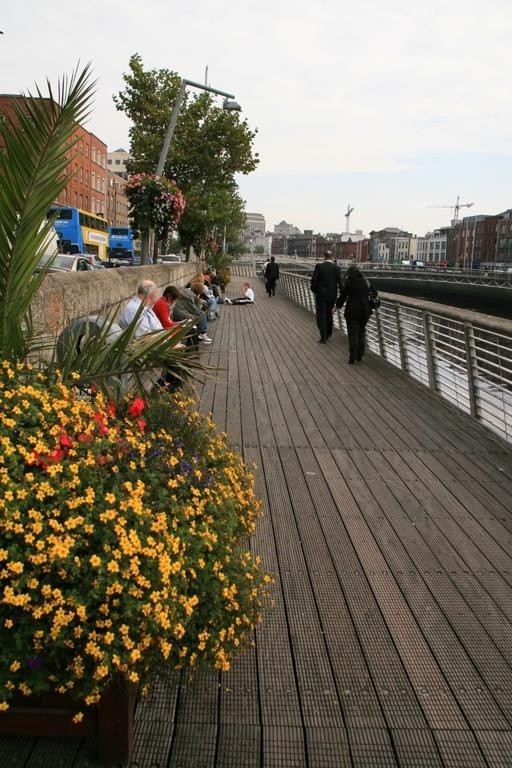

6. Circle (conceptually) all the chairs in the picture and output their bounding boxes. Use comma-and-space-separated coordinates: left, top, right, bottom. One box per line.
225, 298, 233, 304
197, 333, 211, 341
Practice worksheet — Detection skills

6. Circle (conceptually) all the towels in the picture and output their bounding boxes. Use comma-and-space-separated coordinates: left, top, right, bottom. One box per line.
56, 315, 129, 403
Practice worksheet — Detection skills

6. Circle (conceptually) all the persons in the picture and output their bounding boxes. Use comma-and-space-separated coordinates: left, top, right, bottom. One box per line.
186, 269, 224, 322
483, 265, 489, 278
409, 257, 415, 271
264, 255, 279, 297
151, 284, 181, 345
310, 249, 344, 343
332, 264, 374, 364
117, 278, 188, 395
261, 258, 271, 292
332, 257, 337, 265
225, 281, 254, 305
173, 281, 213, 347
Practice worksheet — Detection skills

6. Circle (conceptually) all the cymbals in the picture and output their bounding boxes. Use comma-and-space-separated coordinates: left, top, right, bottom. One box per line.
427, 194, 474, 219
342, 201, 355, 231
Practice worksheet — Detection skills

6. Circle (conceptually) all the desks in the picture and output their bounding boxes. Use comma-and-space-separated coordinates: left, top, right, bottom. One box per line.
154, 78, 242, 180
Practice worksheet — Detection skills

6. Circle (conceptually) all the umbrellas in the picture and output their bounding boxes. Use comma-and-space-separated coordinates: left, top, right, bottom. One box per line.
318, 339, 327, 344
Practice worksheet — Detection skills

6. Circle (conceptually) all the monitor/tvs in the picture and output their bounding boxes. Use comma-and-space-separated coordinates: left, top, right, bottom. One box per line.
174, 317, 197, 328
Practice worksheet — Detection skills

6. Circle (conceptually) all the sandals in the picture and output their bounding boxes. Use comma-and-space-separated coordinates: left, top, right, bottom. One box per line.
108, 226, 142, 259
45, 207, 110, 262
401, 260, 512, 274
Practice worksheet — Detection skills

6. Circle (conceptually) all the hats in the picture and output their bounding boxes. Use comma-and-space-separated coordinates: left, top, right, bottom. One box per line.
29, 252, 182, 276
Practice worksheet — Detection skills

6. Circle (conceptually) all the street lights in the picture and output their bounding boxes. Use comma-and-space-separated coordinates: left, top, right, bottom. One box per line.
368, 286, 381, 308
